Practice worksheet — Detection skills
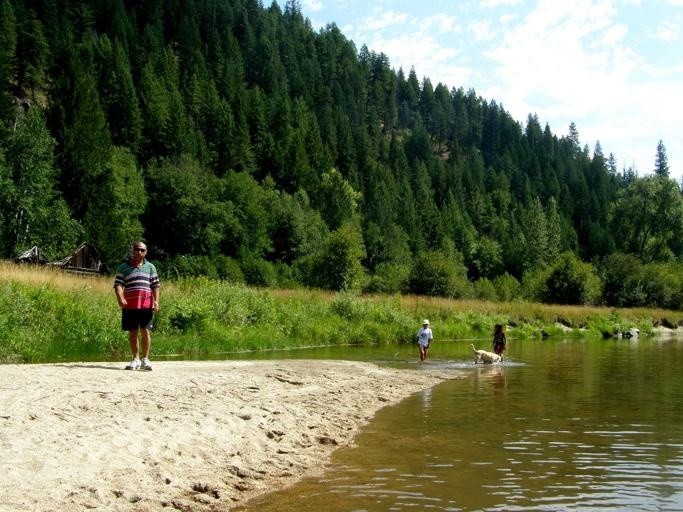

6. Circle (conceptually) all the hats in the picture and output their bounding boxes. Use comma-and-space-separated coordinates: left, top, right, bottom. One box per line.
422, 320, 430, 325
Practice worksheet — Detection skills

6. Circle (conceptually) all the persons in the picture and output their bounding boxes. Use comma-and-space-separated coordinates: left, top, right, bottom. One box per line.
112, 239, 161, 372
415, 320, 433, 361
490, 324, 506, 363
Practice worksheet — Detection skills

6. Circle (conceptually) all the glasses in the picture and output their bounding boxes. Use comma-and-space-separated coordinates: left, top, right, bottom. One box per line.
134, 248, 147, 252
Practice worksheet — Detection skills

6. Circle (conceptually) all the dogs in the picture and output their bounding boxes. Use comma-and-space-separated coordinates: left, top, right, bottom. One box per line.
469, 344, 501, 364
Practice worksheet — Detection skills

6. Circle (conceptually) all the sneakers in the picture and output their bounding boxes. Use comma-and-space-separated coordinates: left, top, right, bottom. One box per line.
126, 358, 142, 370
140, 359, 153, 371
